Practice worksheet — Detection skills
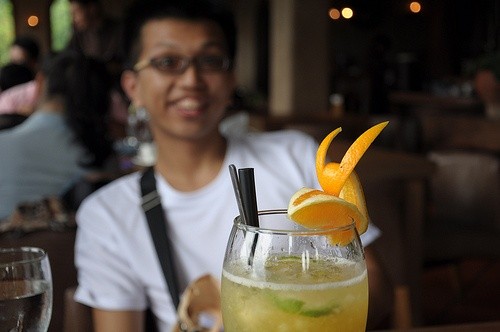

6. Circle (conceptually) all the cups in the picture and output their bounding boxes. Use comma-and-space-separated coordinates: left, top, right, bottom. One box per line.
0, 246, 54, 332
220, 209, 371, 332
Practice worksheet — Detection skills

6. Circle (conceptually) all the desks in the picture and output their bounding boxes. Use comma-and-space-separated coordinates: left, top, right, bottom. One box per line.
393, 91, 483, 155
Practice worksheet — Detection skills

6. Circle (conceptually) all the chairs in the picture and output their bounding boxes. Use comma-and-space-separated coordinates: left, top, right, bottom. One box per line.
319, 139, 433, 332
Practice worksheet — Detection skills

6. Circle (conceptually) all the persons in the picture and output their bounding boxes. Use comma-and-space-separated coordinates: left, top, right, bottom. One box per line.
0, 0, 385, 332
464, 65, 500, 121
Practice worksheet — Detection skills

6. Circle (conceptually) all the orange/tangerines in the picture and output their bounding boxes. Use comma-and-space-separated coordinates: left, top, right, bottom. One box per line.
288, 120, 390, 246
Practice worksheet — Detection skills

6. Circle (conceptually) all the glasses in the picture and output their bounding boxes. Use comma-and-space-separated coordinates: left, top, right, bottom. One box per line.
133, 52, 230, 75
